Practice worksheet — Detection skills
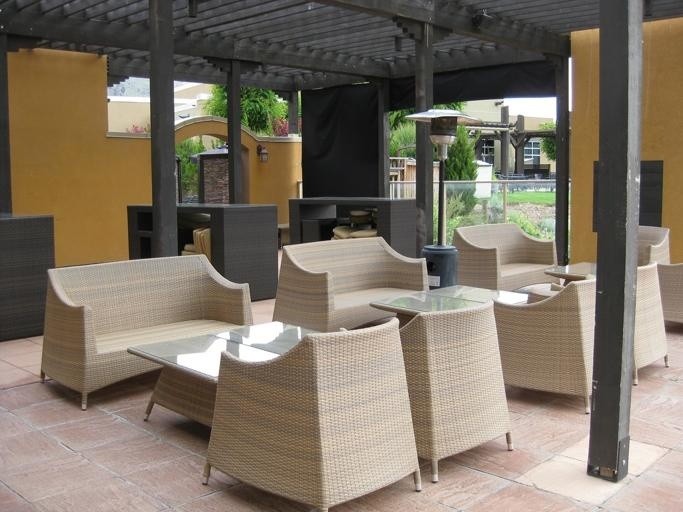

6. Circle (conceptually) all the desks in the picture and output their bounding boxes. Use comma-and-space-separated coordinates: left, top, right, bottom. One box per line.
126, 202, 279, 302
127, 322, 329, 422
288, 197, 419, 257
370, 284, 528, 316
2, 212, 56, 342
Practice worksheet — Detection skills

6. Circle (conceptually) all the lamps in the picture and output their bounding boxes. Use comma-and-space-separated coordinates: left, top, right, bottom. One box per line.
256, 147, 269, 164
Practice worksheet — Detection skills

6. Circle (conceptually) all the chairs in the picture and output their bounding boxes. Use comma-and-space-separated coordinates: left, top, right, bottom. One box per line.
38, 253, 253, 410
656, 262, 682, 325
399, 297, 515, 484
453, 222, 560, 292
493, 261, 670, 415
638, 225, 671, 264
544, 260, 597, 280
271, 235, 428, 333
200, 316, 423, 511
